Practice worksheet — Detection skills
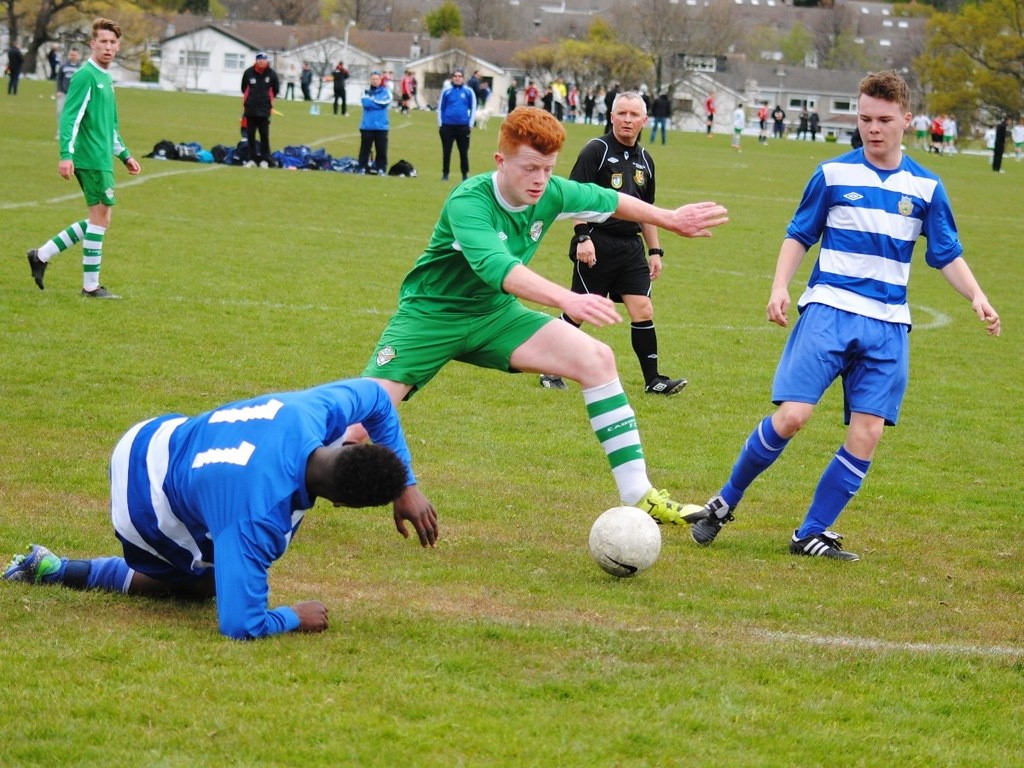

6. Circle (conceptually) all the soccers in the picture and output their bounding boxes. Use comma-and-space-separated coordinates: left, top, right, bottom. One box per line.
588, 506, 661, 577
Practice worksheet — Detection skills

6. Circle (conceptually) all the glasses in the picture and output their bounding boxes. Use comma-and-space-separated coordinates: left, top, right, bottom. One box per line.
454, 74, 463, 78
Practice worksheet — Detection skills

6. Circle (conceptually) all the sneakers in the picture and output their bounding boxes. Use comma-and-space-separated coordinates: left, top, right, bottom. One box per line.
690, 495, 734, 547
619, 488, 711, 524
645, 375, 688, 395
0, 543, 63, 585
539, 373, 567, 389
790, 530, 859, 562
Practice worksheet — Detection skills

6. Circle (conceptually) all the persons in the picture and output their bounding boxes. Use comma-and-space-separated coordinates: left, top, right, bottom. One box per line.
276, 60, 1024, 174
356, 70, 391, 177
331, 106, 709, 523
7, 37, 24, 95
2, 375, 438, 640
48, 42, 59, 79
54, 47, 82, 140
240, 52, 280, 170
26, 17, 140, 301
691, 73, 1000, 562
538, 92, 688, 398
437, 70, 475, 181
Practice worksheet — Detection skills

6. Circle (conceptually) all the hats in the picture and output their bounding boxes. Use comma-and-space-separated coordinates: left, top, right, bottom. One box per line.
453, 67, 464, 74
370, 67, 382, 76
256, 50, 269, 59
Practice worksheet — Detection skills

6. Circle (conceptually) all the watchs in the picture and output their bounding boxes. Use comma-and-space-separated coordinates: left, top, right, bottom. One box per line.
576, 235, 593, 242
648, 248, 664, 257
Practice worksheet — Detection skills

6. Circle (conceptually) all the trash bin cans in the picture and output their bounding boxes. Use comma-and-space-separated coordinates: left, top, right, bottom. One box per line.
825, 136, 837, 143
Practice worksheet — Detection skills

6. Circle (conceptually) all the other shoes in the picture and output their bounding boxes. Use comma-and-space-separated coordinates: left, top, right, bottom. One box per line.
377, 170, 385, 176
462, 174, 468, 181
244, 160, 257, 167
260, 160, 269, 168
357, 167, 366, 174
442, 174, 449, 180
